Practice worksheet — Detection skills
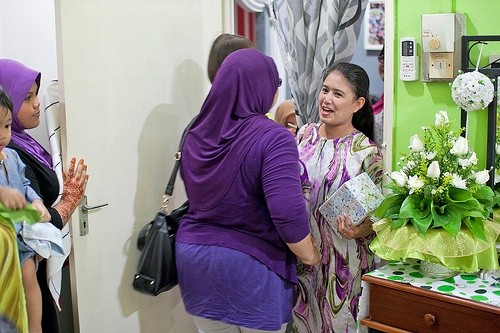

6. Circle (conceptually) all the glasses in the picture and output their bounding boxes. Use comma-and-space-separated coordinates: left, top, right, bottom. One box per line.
277, 77, 282, 87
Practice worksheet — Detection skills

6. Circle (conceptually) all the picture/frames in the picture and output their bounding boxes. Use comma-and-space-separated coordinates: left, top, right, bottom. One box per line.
363, 0, 385, 50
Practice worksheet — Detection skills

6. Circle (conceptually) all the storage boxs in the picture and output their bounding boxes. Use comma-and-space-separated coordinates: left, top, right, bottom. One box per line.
318, 172, 385, 240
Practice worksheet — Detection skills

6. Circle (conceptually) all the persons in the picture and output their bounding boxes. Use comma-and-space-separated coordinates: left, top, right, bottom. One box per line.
285, 62, 383, 333
0, 59, 89, 333
175, 48, 322, 333
207, 33, 254, 84
368, 46, 384, 150
265, 99, 297, 134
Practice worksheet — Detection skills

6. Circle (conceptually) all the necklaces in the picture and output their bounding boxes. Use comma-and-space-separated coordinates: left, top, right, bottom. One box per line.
0, 156, 7, 165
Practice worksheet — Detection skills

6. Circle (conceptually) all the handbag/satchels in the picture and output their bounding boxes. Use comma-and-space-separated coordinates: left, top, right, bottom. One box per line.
132, 113, 199, 296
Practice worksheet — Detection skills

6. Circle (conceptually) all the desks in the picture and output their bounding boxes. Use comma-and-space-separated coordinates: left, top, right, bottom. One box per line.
358, 256, 500, 333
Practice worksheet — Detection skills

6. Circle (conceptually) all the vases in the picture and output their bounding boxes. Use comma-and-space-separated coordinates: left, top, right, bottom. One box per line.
421, 260, 457, 276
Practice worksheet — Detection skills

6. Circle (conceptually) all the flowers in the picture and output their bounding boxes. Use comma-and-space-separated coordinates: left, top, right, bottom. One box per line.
374, 110, 500, 243
